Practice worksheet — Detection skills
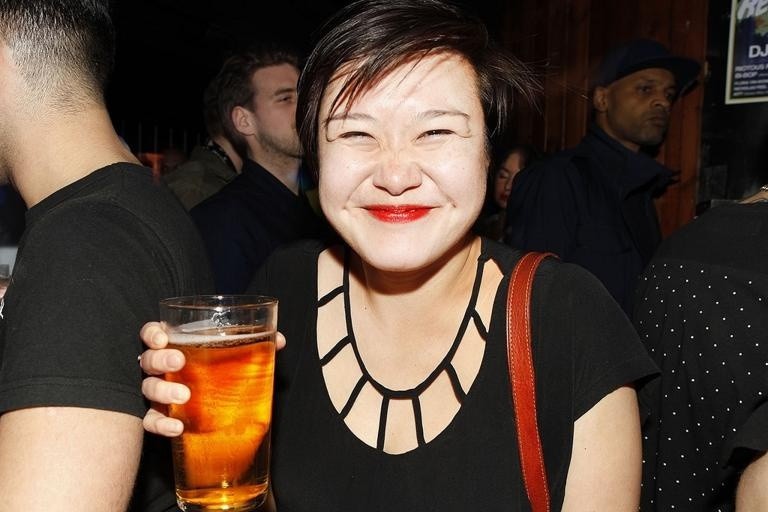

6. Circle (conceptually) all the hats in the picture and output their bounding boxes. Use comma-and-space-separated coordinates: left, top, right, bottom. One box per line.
586, 40, 701, 101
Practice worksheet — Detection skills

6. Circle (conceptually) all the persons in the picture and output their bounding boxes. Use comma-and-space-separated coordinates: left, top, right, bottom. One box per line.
0, 0, 212, 512
473, 144, 541, 243
165, 92, 247, 212
139, 0, 662, 512
506, 38, 702, 309
188, 45, 319, 295
626, 183, 768, 512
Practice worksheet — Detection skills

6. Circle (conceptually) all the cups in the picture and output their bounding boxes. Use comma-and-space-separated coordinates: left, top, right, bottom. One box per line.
160, 295, 278, 511
